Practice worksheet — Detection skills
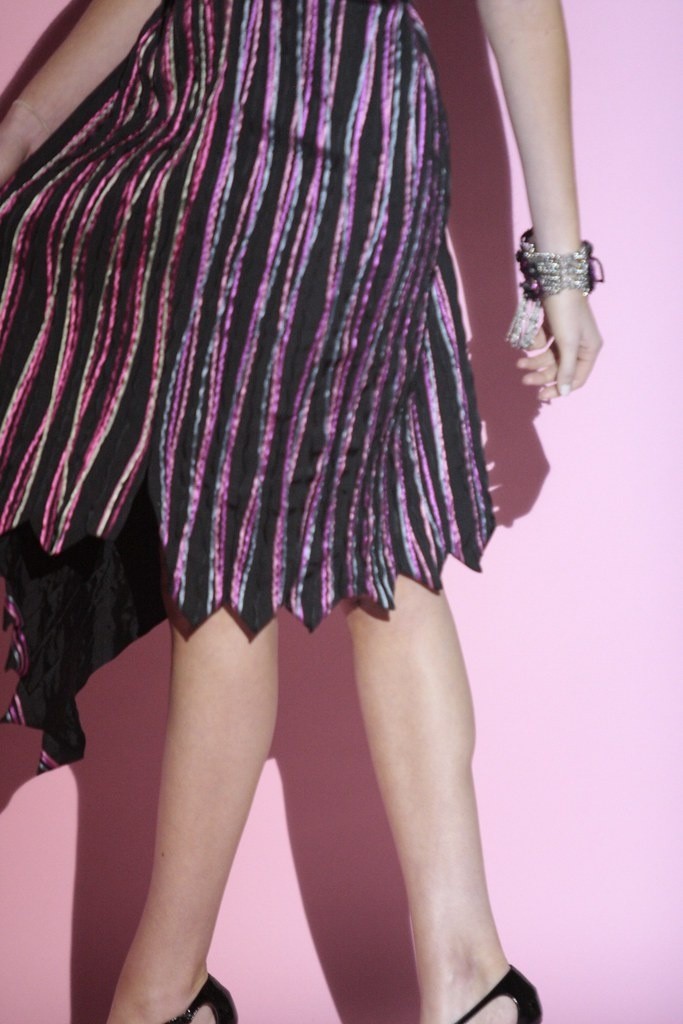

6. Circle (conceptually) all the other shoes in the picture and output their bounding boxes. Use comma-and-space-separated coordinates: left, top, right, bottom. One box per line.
455, 964, 543, 1024
165, 972, 237, 1024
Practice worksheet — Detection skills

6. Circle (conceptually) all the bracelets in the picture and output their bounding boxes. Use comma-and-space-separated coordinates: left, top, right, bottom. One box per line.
507, 228, 604, 349
13, 97, 54, 137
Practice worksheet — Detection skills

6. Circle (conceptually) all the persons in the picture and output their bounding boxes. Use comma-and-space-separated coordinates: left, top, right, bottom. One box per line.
0, 0, 604, 1024
